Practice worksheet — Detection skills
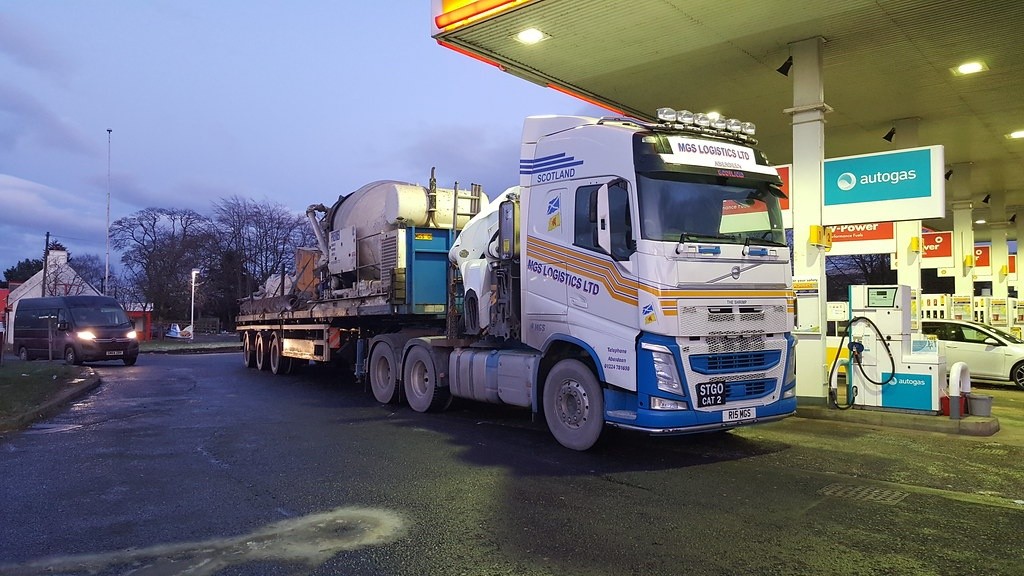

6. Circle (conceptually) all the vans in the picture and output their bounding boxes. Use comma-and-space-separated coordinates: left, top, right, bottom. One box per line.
12, 295, 140, 366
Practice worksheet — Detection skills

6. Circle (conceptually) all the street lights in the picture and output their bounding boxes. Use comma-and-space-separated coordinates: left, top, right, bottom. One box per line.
190, 268, 201, 340
104, 127, 112, 296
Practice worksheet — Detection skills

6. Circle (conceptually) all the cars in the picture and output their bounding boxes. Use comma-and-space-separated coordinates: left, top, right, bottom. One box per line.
826, 317, 850, 376
922, 317, 1024, 391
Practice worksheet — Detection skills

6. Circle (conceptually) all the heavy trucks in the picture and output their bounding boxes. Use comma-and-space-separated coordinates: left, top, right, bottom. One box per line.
233, 107, 802, 453
